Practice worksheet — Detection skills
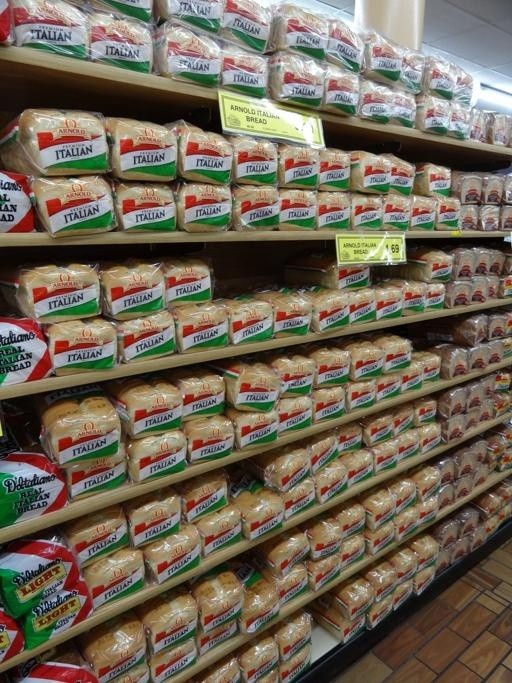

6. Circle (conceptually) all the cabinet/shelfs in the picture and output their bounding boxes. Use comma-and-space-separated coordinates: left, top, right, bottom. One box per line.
2, 46, 512, 680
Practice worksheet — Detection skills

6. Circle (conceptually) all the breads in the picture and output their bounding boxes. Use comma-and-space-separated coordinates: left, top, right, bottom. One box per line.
199, 656, 241, 683
472, 247, 492, 280
233, 136, 277, 185
464, 346, 490, 369
224, 365, 278, 412
181, 476, 231, 521
9, 264, 101, 323
487, 342, 504, 364
386, 476, 417, 510
391, 580, 414, 610
436, 549, 449, 570
459, 204, 478, 230
112, 666, 149, 683
361, 81, 392, 121
318, 192, 351, 230
273, 52, 322, 109
372, 335, 413, 373
309, 346, 350, 387
477, 403, 494, 422
449, 106, 471, 140
1, 321, 51, 381
106, 264, 165, 320
80, 0, 156, 20
0, 455, 65, 525
278, 644, 309, 683
448, 280, 474, 308
301, 435, 340, 473
428, 342, 468, 379
180, 187, 231, 232
498, 449, 512, 472
144, 592, 198, 656
255, 290, 312, 337
391, 90, 417, 129
386, 544, 419, 575
437, 387, 465, 420
299, 513, 343, 561
338, 534, 366, 565
0, 537, 81, 617
361, 558, 397, 592
278, 190, 316, 229
225, 559, 280, 635
223, 0, 271, 38
406, 250, 454, 284
234, 631, 279, 683
413, 397, 437, 427
283, 477, 315, 519
389, 403, 414, 437
184, 420, 235, 462
119, 315, 174, 365
311, 388, 347, 423
82, 622, 147, 680
15, 656, 98, 683
456, 314, 487, 345
448, 537, 468, 564
417, 98, 449, 133
416, 497, 439, 525
413, 350, 441, 383
370, 284, 403, 320
397, 430, 420, 459
440, 417, 463, 441
235, 487, 282, 542
453, 171, 480, 203
39, 394, 121, 467
473, 111, 487, 143
168, 263, 211, 306
260, 667, 279, 683
24, 583, 94, 650
376, 375, 401, 400
430, 455, 455, 484
324, 573, 374, 622
369, 442, 399, 475
474, 491, 503, 516
411, 198, 438, 231
480, 514, 501, 534
340, 290, 375, 325
29, 179, 116, 238
251, 444, 310, 494
479, 206, 500, 230
358, 412, 394, 447
197, 510, 242, 557
307, 600, 365, 644
303, 554, 342, 591
163, 27, 221, 84
342, 451, 374, 485
465, 382, 486, 411
399, 366, 421, 392
269, 609, 311, 662
324, 65, 361, 114
411, 162, 451, 196
407, 532, 440, 560
382, 277, 427, 316
430, 518, 459, 546
502, 206, 512, 231
4, 1, 89, 62
353, 195, 381, 230
319, 148, 350, 192
175, 306, 229, 351
230, 410, 277, 451
493, 423, 511, 449
47, 321, 118, 374
453, 65, 474, 104
486, 274, 500, 300
330, 424, 361, 458
465, 530, 486, 551
328, 17, 364, 71
130, 431, 188, 481
129, 493, 185, 546
92, 14, 155, 73
487, 312, 507, 341
278, 143, 319, 189
393, 507, 418, 541
425, 58, 453, 97
451, 248, 476, 281
361, 489, 397, 530
498, 503, 512, 521
149, 641, 197, 682
362, 32, 401, 84
453, 505, 479, 532
271, 355, 314, 398
275, 398, 312, 432
222, 299, 276, 344
473, 464, 488, 485
160, 0, 221, 25
436, 484, 454, 507
481, 173, 504, 205
86, 550, 147, 610
435, 199, 461, 231
351, 152, 391, 194
234, 187, 277, 231
68, 509, 129, 569
177, 376, 224, 417
344, 381, 376, 412
491, 478, 512, 501
414, 566, 436, 594
167, 123, 231, 184
492, 394, 512, 418
468, 436, 488, 462
418, 423, 442, 454
340, 340, 385, 380
260, 526, 309, 577
106, 118, 178, 183
146, 529, 204, 584
366, 598, 391, 629
273, 0, 327, 59
331, 500, 366, 538
463, 411, 477, 430
489, 249, 506, 276
424, 282, 446, 310
451, 446, 476, 478
191, 569, 244, 631
490, 112, 512, 146
494, 371, 511, 393
199, 621, 238, 654
454, 475, 472, 496
405, 463, 442, 500
120, 383, 181, 437
0, 606, 22, 660
4, 107, 109, 177
71, 454, 128, 493
317, 259, 372, 290
0, 173, 35, 233
254, 558, 309, 603
484, 434, 504, 459
315, 462, 348, 506
221, 44, 268, 96
118, 185, 177, 232
383, 196, 410, 231
365, 523, 395, 554
292, 286, 348, 334
382, 153, 415, 195
398, 51, 422, 93
470, 277, 487, 304
